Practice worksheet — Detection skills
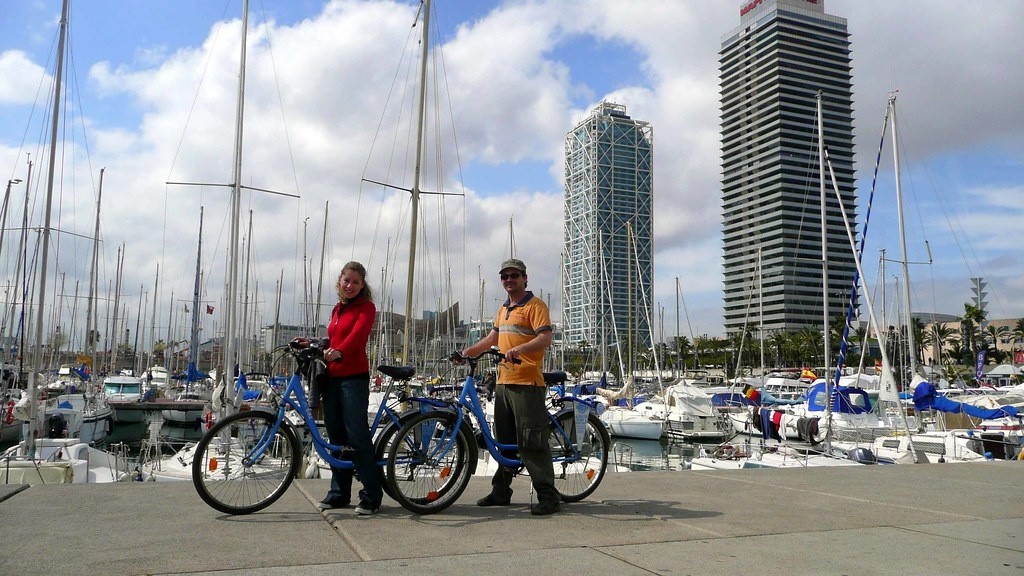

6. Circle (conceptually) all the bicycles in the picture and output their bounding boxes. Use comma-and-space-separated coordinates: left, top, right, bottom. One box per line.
382, 346, 609, 517
191, 339, 464, 516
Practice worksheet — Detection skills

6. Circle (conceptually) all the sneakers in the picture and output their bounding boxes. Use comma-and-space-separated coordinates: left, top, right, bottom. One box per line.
531, 494, 561, 515
354, 499, 382, 515
317, 493, 351, 508
477, 492, 511, 506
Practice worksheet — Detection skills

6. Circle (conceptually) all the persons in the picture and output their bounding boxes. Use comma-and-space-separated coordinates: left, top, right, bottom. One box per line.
61, 381, 76, 395
452, 258, 561, 515
290, 261, 385, 514
49, 414, 64, 438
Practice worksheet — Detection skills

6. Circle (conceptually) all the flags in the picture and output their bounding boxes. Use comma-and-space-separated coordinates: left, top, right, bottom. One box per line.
977, 379, 998, 393
800, 368, 817, 385
1015, 351, 1024, 362
186, 305, 190, 313
876, 360, 882, 372
207, 305, 215, 314
743, 384, 759, 402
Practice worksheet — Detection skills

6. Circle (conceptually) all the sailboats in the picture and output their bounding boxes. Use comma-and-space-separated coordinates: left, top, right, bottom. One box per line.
1, 0, 1024, 488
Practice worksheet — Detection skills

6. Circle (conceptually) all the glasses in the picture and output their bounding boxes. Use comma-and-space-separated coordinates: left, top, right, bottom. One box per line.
501, 273, 522, 280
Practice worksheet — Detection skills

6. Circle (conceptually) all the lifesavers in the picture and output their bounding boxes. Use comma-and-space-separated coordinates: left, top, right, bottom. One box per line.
205, 411, 213, 430
6, 401, 17, 424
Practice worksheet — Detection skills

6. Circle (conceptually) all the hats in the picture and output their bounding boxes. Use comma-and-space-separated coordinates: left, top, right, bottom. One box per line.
499, 259, 526, 274
